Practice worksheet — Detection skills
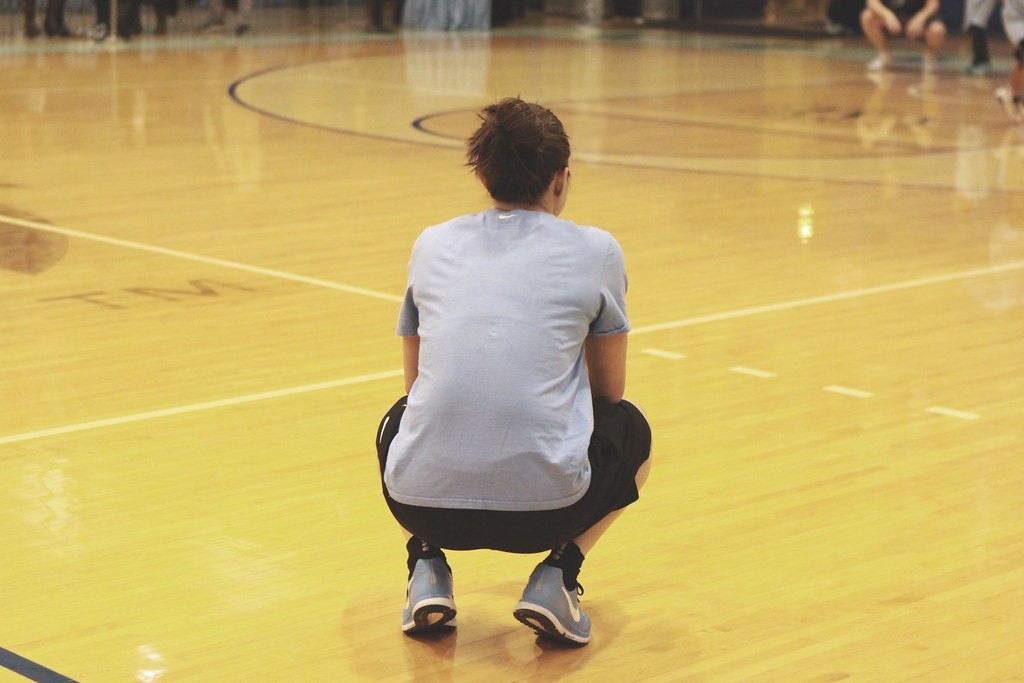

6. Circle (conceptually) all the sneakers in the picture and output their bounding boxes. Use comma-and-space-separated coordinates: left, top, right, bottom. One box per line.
401, 553, 457, 634
513, 562, 591, 644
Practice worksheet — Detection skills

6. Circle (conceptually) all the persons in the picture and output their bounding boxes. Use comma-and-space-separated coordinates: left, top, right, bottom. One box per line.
961, 0, 998, 78
859, 0, 946, 75
997, 0, 1024, 127
376, 96, 656, 648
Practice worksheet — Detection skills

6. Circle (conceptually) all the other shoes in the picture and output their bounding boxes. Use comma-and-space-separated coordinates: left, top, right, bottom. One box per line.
867, 54, 893, 71
88, 21, 111, 40
960, 59, 992, 82
922, 52, 938, 73
23, 22, 40, 38
195, 19, 229, 32
366, 22, 391, 34
154, 21, 167, 36
236, 18, 254, 36
118, 21, 130, 40
44, 23, 73, 38
995, 85, 1022, 124
